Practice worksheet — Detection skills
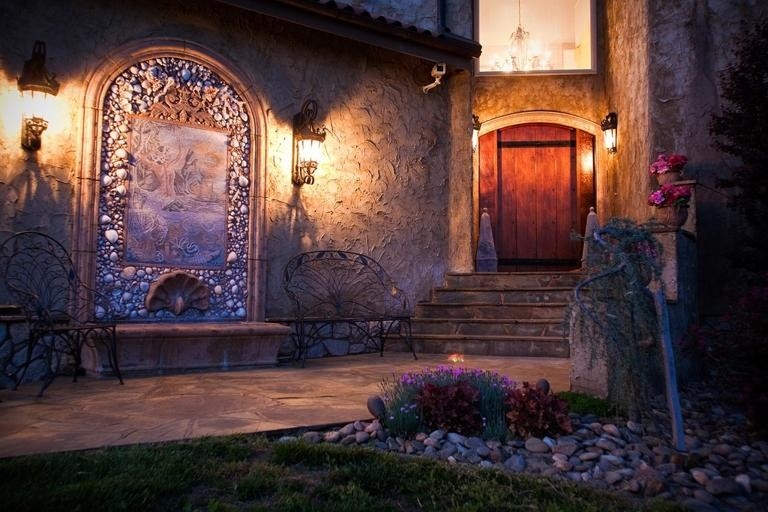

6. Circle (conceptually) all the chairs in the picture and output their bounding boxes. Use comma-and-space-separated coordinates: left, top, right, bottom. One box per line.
0, 230, 124, 396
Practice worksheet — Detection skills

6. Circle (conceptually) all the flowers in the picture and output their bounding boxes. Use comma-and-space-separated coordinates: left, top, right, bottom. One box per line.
648, 153, 689, 177
646, 182, 693, 210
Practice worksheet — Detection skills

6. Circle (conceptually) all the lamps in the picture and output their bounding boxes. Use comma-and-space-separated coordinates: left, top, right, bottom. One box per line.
290, 99, 326, 186
494, 0, 555, 71
600, 112, 617, 154
17, 38, 61, 153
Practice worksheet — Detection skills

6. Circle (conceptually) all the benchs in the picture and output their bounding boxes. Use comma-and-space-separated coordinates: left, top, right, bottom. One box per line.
282, 249, 418, 370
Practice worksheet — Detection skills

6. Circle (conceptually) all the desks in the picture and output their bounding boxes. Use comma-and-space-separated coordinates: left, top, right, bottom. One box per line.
0, 314, 70, 391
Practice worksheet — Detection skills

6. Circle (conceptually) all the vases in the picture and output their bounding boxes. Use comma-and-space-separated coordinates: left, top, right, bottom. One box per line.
653, 204, 691, 232
655, 167, 684, 187
